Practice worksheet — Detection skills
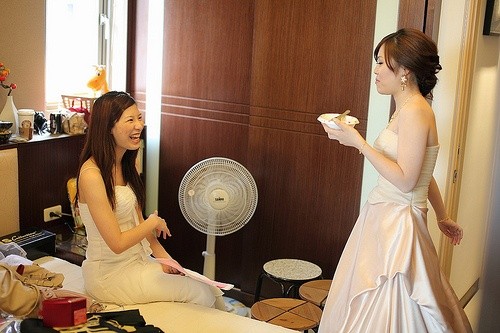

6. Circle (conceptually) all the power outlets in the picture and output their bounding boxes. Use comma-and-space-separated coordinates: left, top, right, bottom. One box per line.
44, 205, 61, 222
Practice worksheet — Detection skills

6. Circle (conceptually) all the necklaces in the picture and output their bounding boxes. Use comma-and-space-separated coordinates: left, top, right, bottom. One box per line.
387, 92, 418, 125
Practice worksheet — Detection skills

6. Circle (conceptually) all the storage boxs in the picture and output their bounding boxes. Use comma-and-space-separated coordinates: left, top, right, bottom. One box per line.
42, 297, 87, 327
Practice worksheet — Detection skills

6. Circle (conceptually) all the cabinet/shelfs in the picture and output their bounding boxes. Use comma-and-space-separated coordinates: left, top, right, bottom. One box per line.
0, 127, 91, 243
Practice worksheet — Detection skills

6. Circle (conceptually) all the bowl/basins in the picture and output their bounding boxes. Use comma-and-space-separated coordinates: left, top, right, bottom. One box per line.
317, 113, 359, 129
0, 122, 13, 142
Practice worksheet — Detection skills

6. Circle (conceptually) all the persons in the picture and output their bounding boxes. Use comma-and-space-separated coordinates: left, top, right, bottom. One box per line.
317, 26, 474, 333
74, 91, 224, 310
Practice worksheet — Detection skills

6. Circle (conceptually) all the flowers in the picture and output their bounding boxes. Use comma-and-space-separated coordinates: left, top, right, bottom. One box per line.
0, 62, 17, 96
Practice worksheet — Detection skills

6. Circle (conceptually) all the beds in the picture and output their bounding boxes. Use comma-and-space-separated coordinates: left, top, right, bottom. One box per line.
0, 229, 303, 333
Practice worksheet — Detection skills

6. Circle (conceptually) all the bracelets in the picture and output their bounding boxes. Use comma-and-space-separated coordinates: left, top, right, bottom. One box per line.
358, 141, 366, 154
436, 217, 450, 223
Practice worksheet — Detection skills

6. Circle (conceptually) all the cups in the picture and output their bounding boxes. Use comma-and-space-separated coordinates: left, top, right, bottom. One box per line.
18, 109, 35, 135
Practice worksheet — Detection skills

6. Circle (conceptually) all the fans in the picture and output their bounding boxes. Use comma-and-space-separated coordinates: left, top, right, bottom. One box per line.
177, 157, 258, 283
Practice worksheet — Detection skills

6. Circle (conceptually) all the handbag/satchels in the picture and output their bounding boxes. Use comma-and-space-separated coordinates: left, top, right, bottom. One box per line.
21, 309, 165, 333
69, 98, 91, 133
1, 254, 65, 318
58, 107, 86, 136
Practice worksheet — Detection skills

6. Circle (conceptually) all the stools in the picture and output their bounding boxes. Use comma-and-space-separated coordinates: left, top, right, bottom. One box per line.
254, 258, 324, 303
299, 280, 332, 308
250, 298, 323, 333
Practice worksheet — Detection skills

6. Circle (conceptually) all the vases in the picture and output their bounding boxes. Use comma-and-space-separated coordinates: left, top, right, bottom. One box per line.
0, 96, 18, 136
